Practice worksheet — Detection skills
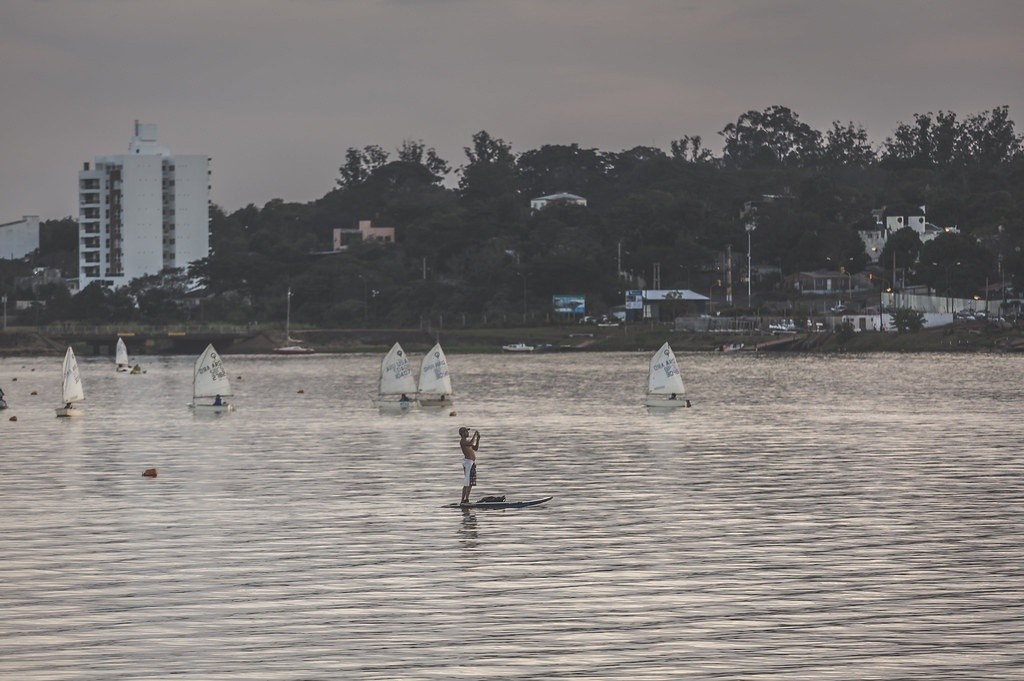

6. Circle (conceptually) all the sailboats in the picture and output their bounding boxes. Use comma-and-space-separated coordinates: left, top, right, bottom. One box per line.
116, 337, 142, 374
273, 288, 315, 353
187, 343, 234, 412
416, 343, 453, 406
372, 342, 420, 409
645, 341, 692, 407
55, 346, 86, 418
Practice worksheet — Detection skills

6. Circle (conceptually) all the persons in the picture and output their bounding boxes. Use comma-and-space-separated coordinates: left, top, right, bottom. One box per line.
213, 395, 223, 405
441, 394, 445, 401
399, 394, 409, 402
459, 427, 481, 502
64, 403, 72, 409
669, 393, 677, 400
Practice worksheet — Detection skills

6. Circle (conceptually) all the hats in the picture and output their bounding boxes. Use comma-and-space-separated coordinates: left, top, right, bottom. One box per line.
459, 427, 470, 434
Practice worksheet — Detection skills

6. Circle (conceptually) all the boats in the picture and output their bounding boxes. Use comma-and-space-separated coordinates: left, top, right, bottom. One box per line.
715, 342, 744, 352
502, 342, 534, 352
